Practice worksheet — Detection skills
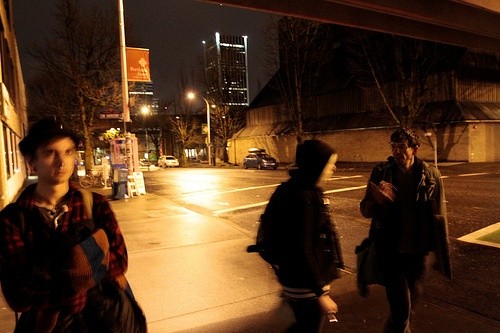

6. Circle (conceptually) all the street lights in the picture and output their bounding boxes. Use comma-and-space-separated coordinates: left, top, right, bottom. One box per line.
188, 92, 211, 164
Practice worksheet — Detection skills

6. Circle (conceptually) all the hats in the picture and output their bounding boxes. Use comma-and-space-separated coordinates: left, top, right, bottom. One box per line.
18, 115, 80, 158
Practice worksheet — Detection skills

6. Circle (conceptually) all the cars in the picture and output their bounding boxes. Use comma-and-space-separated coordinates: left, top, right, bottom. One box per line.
158, 156, 179, 166
139, 161, 150, 167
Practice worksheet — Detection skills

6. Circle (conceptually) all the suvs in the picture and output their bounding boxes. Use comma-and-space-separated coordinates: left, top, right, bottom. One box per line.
243, 148, 278, 169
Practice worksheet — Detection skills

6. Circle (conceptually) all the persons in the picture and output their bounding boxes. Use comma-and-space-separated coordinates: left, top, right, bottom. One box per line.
0, 124, 148, 333
353, 124, 452, 332
243, 139, 344, 332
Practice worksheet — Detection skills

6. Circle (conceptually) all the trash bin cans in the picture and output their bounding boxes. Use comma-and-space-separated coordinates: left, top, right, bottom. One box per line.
109, 165, 130, 198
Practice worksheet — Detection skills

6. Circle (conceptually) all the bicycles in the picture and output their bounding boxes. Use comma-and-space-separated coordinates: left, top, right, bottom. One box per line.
79, 168, 112, 188
